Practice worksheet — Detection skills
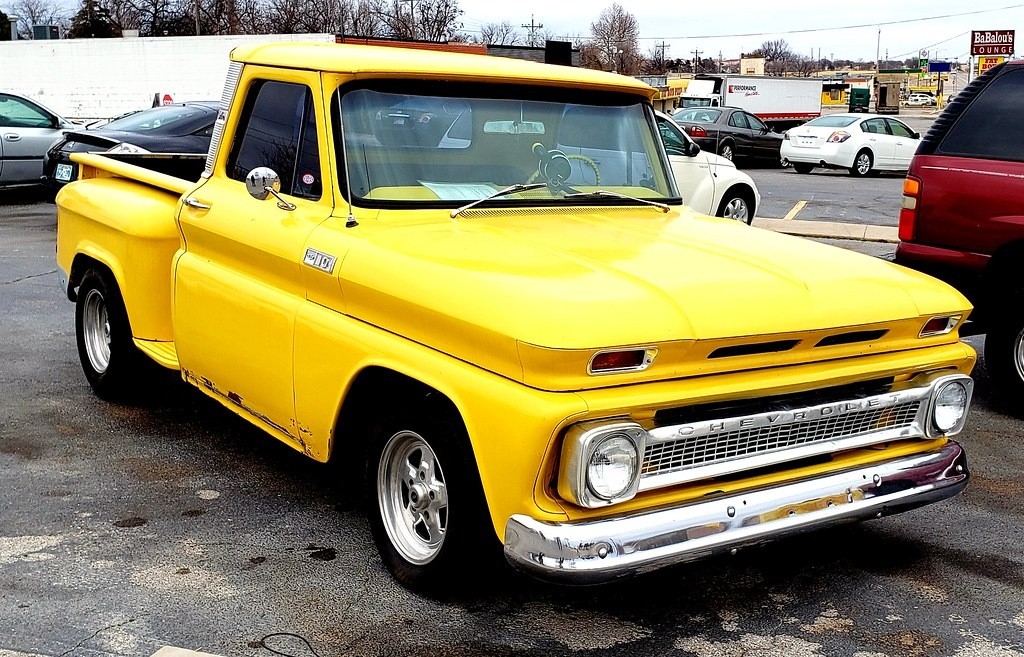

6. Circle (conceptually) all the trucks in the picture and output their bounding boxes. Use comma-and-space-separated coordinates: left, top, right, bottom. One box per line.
848, 88, 871, 113
667, 72, 823, 136
875, 82, 900, 114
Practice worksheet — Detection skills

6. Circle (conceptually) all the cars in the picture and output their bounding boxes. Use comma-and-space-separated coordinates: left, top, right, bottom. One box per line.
0, 91, 98, 194
435, 102, 761, 228
779, 112, 925, 178
373, 95, 468, 148
656, 106, 786, 170
40, 100, 378, 207
896, 58, 1024, 420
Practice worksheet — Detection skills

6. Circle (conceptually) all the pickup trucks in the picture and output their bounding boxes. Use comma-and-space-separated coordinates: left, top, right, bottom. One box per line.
53, 42, 975, 597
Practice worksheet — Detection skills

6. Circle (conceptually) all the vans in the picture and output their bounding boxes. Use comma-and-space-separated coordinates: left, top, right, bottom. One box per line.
901, 94, 937, 106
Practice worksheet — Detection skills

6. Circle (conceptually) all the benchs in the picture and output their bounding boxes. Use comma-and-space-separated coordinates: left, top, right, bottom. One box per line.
351, 162, 524, 197
